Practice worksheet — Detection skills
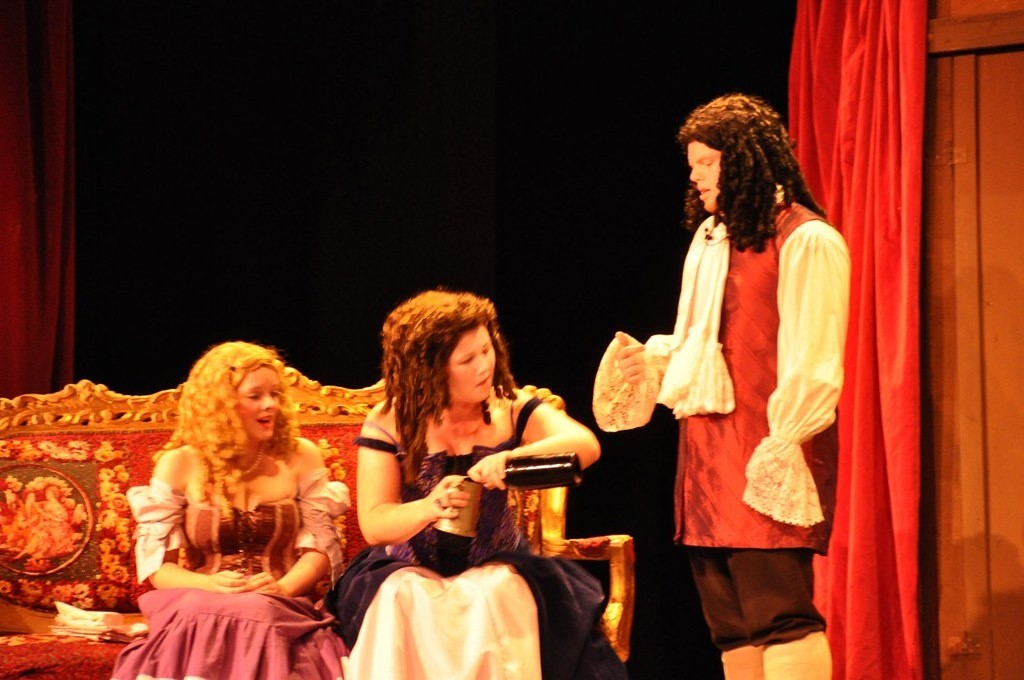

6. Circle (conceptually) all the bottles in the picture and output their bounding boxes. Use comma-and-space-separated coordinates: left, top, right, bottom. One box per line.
463, 452, 582, 487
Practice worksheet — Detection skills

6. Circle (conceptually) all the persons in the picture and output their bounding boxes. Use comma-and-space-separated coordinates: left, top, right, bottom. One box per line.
108, 340, 350, 680
323, 291, 630, 680
592, 87, 853, 680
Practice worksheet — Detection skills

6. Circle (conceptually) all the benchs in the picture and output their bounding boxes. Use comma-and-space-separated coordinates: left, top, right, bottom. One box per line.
0, 366, 636, 680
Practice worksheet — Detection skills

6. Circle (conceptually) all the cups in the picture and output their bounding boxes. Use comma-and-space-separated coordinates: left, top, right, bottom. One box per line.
431, 477, 482, 537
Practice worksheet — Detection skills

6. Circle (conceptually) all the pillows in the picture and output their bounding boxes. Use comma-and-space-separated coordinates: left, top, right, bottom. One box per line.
0, 438, 139, 613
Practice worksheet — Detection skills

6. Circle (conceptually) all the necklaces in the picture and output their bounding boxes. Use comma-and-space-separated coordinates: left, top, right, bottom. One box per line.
243, 444, 265, 477
